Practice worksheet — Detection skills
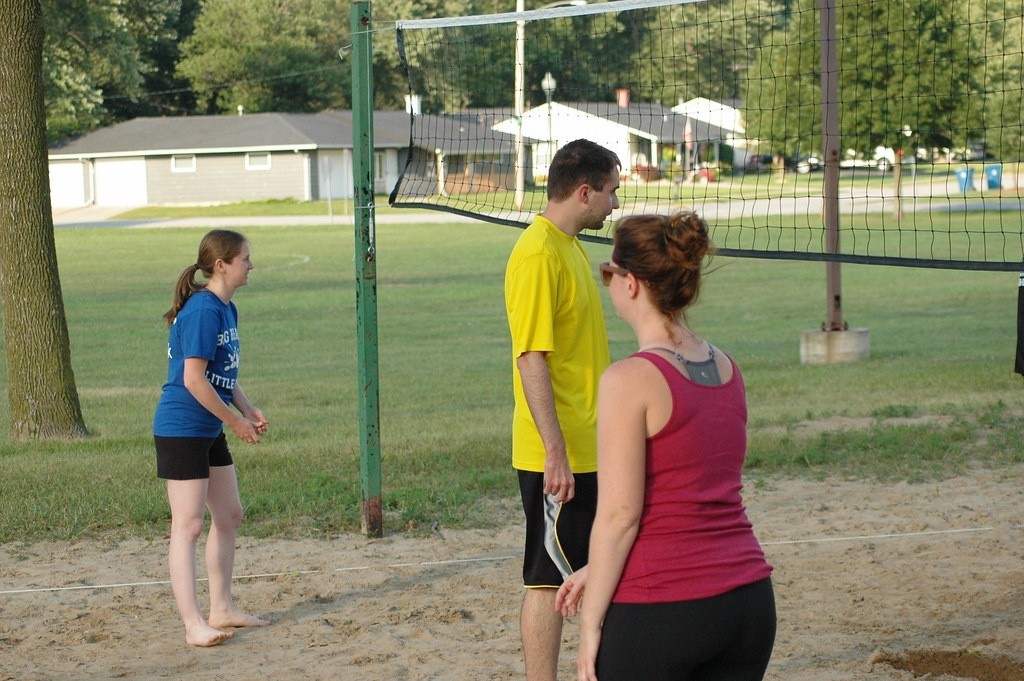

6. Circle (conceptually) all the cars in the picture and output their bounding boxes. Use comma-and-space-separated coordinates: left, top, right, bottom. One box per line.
749, 148, 818, 175
838, 146, 917, 173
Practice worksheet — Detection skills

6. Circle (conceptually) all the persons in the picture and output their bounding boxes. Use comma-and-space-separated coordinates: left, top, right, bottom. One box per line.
553, 211, 778, 681
503, 140, 624, 681
152, 230, 269, 650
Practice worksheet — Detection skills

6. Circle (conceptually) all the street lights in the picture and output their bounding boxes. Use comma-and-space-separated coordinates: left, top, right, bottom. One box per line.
540, 72, 557, 166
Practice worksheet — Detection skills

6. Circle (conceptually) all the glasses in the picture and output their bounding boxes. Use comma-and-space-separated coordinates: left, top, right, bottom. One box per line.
600, 262, 651, 288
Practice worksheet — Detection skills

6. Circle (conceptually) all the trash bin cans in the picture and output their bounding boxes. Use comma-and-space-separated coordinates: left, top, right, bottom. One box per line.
985, 165, 1002, 189
955, 167, 974, 192
